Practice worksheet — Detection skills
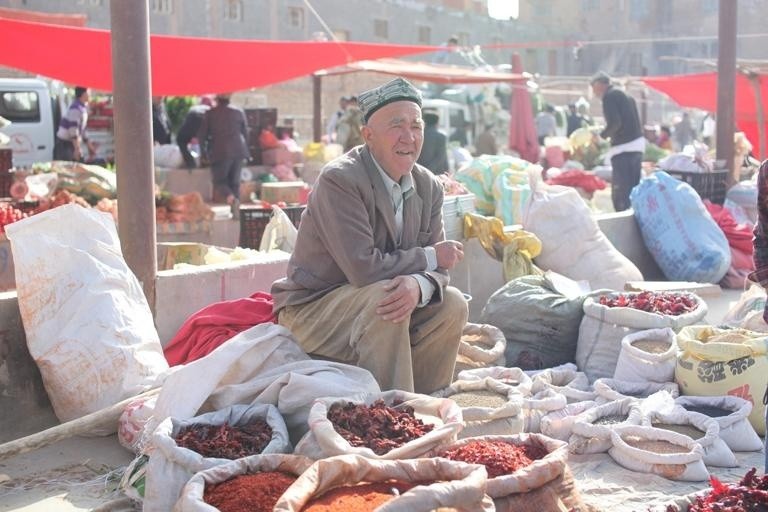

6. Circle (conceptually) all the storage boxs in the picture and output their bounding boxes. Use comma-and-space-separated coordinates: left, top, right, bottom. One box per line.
259, 178, 310, 204
155, 239, 209, 271
434, 193, 481, 246
238, 178, 260, 201
238, 103, 304, 169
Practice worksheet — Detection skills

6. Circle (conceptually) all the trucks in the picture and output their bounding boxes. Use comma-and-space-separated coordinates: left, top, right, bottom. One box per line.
0, 75, 116, 174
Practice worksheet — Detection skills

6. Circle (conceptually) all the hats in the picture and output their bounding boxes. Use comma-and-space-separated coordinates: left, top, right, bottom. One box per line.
356, 76, 424, 124
589, 72, 609, 86
422, 102, 439, 119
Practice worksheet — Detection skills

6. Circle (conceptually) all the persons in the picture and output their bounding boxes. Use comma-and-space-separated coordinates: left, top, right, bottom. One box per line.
749, 159, 768, 475
